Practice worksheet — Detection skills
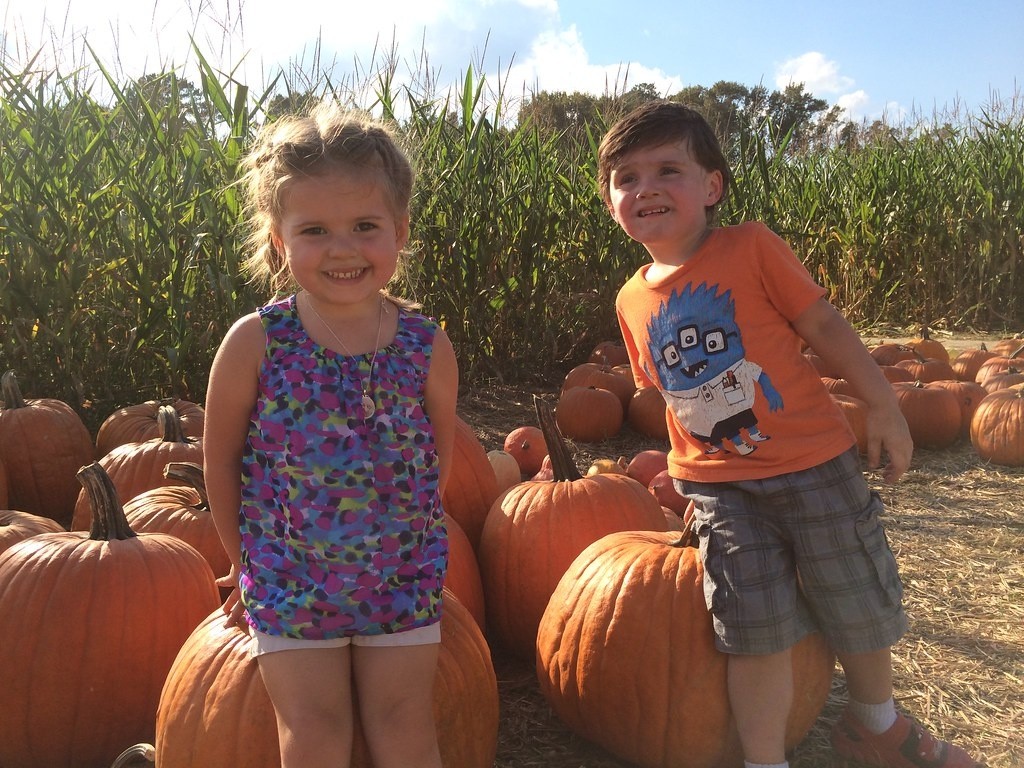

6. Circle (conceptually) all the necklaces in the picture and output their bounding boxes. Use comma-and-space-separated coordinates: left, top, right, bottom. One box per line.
311, 292, 389, 420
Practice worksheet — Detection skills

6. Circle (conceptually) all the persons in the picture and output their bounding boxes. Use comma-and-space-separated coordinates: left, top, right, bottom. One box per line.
599, 99, 973, 768
202, 111, 459, 768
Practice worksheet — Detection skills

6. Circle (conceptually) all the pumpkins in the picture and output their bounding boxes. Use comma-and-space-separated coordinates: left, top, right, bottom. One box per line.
554, 325, 1024, 465
0, 370, 836, 768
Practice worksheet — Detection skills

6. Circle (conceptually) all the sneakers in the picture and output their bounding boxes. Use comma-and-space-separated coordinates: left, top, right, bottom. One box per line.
831, 706, 973, 768
